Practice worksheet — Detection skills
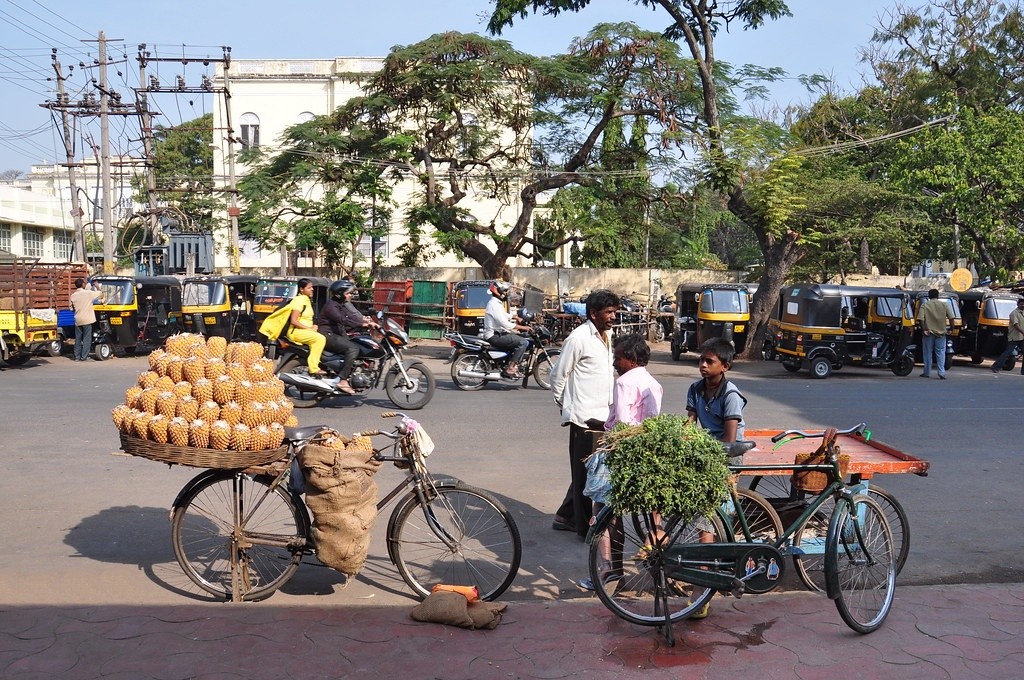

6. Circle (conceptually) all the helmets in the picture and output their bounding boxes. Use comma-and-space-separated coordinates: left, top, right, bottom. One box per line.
329, 280, 354, 302
490, 280, 510, 301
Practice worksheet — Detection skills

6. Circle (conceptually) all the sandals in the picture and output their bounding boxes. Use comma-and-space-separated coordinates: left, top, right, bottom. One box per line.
312, 370, 327, 375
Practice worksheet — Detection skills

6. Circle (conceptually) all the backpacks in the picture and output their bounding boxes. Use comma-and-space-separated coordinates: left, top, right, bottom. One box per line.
273, 298, 307, 338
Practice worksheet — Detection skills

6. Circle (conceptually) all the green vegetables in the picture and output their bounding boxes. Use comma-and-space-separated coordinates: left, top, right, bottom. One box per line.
601, 414, 735, 524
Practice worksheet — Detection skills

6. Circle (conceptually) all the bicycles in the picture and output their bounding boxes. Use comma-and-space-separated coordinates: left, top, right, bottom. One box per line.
585, 421, 897, 649
168, 412, 522, 604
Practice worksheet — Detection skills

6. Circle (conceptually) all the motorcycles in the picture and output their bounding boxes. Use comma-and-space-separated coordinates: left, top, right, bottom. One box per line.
88, 274, 184, 361
543, 282, 752, 371
745, 282, 1024, 379
250, 275, 335, 361
272, 306, 436, 410
442, 308, 562, 391
452, 279, 509, 337
179, 275, 259, 344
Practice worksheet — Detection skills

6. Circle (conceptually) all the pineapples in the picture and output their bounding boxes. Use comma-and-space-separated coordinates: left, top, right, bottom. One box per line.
320, 431, 372, 453
112, 332, 301, 453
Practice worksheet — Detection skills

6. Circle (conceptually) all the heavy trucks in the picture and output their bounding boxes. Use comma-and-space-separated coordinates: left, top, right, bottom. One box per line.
0, 257, 88, 370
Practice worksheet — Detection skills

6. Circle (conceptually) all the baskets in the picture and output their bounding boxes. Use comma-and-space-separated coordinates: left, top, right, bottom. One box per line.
120, 431, 290, 470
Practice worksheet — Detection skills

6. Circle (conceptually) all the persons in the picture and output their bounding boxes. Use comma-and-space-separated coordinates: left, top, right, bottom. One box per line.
286, 278, 327, 375
580, 334, 664, 589
916, 289, 955, 380
686, 338, 748, 618
317, 276, 382, 394
69, 279, 103, 362
483, 280, 534, 378
550, 290, 620, 537
990, 299, 1024, 375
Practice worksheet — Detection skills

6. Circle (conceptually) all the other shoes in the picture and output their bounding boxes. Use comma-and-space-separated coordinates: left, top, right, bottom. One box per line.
75, 358, 79, 362
991, 365, 999, 373
83, 356, 91, 361
500, 370, 526, 378
552, 514, 578, 531
920, 374, 929, 378
578, 577, 597, 589
938, 374, 946, 380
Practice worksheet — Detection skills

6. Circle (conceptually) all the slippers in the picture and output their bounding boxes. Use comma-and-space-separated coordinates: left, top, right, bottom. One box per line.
687, 600, 709, 617
337, 384, 354, 394
631, 545, 654, 560
